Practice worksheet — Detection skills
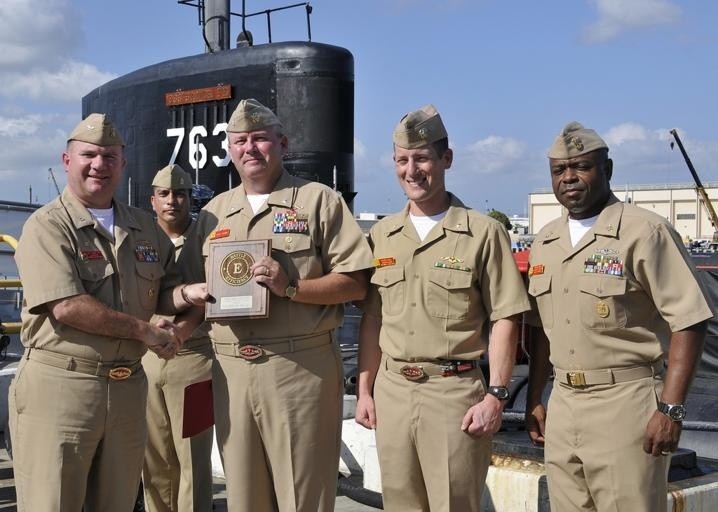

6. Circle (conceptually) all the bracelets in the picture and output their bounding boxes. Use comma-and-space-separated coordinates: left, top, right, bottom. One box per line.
180, 281, 193, 306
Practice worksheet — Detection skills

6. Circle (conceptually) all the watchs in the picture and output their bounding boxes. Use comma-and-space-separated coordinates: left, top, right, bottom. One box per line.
658, 401, 687, 422
486, 385, 512, 401
285, 279, 297, 302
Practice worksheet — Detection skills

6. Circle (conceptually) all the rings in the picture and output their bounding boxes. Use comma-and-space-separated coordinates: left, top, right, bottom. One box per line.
265, 267, 270, 276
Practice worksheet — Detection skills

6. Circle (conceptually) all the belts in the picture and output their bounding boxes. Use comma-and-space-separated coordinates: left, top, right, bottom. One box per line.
182, 337, 211, 349
208, 330, 335, 362
552, 358, 666, 387
23, 347, 143, 382
380, 352, 476, 382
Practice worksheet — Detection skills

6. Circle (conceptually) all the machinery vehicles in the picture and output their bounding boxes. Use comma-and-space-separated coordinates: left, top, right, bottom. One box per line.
667, 127, 717, 254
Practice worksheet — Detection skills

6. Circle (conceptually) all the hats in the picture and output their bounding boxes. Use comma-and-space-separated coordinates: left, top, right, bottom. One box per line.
66, 111, 125, 149
226, 97, 284, 133
546, 120, 609, 158
151, 164, 192, 191
392, 103, 448, 150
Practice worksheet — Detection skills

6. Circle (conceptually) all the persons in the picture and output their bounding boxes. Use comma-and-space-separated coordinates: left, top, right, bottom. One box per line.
136, 165, 213, 512
8, 113, 209, 512
357, 104, 533, 512
144, 98, 378, 512
522, 119, 708, 512
